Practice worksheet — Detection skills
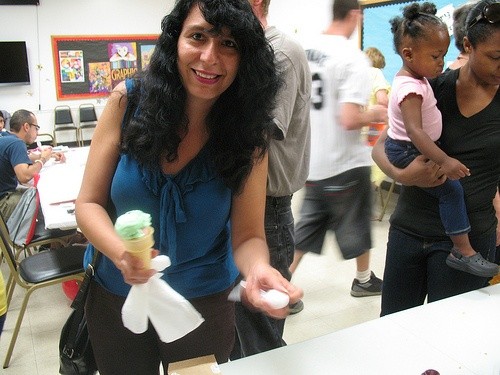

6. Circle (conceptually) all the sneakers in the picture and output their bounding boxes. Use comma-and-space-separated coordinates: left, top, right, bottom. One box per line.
350, 271, 384, 297
287, 297, 304, 315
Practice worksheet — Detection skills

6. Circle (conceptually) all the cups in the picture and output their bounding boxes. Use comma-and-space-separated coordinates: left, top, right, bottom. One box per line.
367, 121, 386, 146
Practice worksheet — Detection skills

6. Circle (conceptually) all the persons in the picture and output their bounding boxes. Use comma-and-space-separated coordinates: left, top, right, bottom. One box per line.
75, 0, 303, 375
372, 0, 500, 317
229, 0, 500, 362
0, 110, 88, 252
384, 3, 500, 278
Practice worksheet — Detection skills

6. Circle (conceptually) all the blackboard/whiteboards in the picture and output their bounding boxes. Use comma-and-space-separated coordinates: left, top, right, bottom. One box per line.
54, 36, 160, 98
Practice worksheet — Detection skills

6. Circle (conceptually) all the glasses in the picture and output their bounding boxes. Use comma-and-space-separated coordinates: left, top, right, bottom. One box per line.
30, 122, 40, 132
467, 2, 500, 30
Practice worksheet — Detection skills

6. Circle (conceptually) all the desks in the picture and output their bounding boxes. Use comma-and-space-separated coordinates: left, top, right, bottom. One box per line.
219, 283, 500, 375
33, 146, 91, 229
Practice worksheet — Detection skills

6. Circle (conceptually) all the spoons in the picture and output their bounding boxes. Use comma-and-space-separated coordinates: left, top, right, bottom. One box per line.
240, 280, 291, 310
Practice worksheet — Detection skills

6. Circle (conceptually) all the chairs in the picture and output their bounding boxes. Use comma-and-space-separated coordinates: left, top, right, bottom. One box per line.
77, 103, 98, 147
0, 207, 87, 369
53, 105, 79, 147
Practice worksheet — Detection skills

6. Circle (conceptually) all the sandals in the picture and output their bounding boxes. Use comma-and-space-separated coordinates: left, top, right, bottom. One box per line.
445, 247, 500, 278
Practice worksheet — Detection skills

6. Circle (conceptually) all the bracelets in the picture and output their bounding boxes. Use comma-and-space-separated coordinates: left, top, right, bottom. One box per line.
34, 159, 44, 168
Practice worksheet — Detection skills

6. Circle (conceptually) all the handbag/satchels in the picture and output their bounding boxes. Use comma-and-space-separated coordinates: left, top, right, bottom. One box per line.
58, 308, 97, 375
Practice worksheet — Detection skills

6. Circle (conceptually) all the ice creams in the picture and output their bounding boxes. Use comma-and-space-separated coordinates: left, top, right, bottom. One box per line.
116, 209, 154, 270
